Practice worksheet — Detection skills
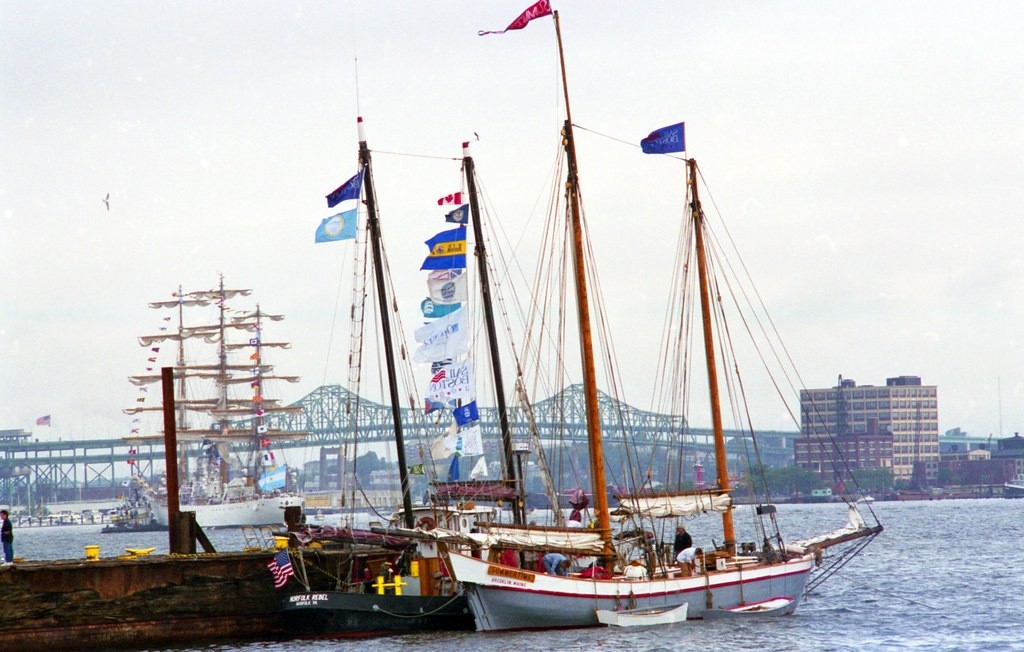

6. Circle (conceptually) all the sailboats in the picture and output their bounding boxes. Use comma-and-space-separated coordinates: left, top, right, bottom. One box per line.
275, 0, 651, 639
122, 275, 307, 530
447, 157, 884, 634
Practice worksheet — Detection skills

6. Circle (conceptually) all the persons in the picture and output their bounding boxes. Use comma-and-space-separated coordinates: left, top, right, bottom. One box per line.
676, 547, 703, 578
542, 553, 571, 577
0, 510, 14, 567
674, 524, 692, 559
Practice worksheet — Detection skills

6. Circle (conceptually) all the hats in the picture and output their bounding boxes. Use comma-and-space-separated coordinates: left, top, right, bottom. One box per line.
675, 525, 684, 529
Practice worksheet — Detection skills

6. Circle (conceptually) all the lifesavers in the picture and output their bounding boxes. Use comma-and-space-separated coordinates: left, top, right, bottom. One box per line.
614, 530, 653, 540
416, 517, 436, 530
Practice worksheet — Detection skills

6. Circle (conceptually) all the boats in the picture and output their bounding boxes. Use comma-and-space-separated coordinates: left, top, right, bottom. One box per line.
755, 504, 777, 515
856, 495, 875, 504
1002, 482, 1024, 500
108, 488, 153, 527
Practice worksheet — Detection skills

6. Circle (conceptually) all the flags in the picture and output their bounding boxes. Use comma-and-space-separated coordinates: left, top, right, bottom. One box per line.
419, 140, 489, 484
641, 123, 685, 154
36, 415, 51, 426
127, 289, 277, 472
315, 209, 356, 243
325, 167, 364, 207
266, 550, 295, 586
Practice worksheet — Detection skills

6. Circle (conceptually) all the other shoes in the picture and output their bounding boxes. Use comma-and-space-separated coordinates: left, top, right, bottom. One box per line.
1, 562, 13, 567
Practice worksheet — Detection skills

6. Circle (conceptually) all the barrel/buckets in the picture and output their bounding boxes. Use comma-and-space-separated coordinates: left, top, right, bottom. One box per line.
275, 537, 290, 548
410, 561, 419, 576
84, 545, 100, 561
